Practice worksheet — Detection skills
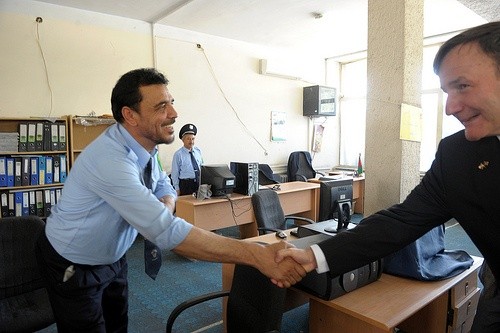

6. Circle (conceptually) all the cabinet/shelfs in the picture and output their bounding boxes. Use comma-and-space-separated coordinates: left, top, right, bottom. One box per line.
0, 114, 117, 223
223, 221, 485, 333
302, 84, 336, 117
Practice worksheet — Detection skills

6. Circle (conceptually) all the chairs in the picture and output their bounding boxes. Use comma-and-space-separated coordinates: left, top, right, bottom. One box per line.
287, 151, 325, 183
0, 215, 62, 333
165, 240, 286, 333
251, 189, 315, 236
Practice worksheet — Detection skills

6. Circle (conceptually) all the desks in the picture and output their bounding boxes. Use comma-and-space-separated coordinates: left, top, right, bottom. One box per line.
175, 168, 365, 264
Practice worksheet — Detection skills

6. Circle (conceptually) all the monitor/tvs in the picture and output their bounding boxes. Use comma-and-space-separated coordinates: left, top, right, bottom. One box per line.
199, 165, 236, 197
318, 178, 354, 232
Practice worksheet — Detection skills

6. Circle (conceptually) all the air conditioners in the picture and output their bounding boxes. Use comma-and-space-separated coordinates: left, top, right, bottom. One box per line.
258, 57, 303, 80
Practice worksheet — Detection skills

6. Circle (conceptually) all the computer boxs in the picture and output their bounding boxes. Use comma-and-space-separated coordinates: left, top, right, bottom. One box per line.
284, 233, 384, 300
229, 161, 260, 197
298, 219, 358, 238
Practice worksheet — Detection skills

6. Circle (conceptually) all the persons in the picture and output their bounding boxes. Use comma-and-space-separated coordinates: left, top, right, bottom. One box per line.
269, 21, 500, 333
34, 67, 308, 333
170, 123, 205, 196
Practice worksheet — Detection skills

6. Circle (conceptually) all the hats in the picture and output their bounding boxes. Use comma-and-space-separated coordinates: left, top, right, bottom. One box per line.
179, 124, 197, 139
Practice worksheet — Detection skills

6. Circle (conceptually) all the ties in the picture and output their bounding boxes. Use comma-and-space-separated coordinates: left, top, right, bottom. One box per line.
189, 151, 200, 184
144, 159, 162, 280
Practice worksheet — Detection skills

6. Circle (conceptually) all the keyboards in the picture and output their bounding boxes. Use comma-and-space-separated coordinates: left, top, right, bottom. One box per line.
290, 228, 298, 236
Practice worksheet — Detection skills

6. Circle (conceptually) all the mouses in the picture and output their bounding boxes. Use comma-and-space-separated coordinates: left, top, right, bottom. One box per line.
277, 231, 287, 238
